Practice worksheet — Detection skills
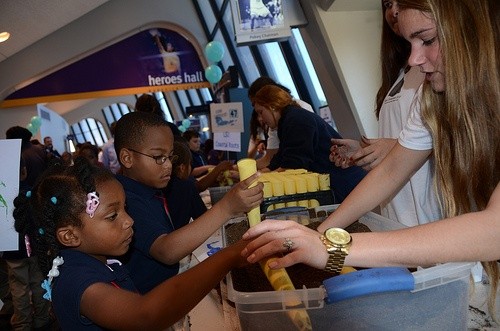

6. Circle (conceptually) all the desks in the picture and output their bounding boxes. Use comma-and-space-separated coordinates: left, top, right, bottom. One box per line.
172, 213, 500, 331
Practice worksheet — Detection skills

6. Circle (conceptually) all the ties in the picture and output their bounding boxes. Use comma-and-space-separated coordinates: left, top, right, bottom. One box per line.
153, 192, 175, 232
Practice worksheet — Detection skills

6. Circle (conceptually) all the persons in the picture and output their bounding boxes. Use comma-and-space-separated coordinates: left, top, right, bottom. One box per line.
241, 0, 500, 331
0, 78, 368, 331
329, 0, 444, 228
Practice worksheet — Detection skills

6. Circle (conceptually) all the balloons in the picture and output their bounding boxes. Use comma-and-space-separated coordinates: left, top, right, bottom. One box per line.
205, 65, 222, 83
205, 40, 225, 62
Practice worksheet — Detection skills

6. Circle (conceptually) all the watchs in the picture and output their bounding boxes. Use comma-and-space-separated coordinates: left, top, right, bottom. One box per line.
319, 227, 352, 276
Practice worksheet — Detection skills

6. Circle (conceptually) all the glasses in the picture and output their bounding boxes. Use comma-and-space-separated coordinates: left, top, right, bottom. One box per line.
126, 148, 173, 165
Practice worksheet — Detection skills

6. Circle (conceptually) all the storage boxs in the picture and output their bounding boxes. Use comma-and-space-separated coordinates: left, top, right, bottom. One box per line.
222, 204, 477, 331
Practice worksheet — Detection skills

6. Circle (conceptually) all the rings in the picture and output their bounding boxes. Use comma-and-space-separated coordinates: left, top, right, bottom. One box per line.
283, 237, 295, 250
